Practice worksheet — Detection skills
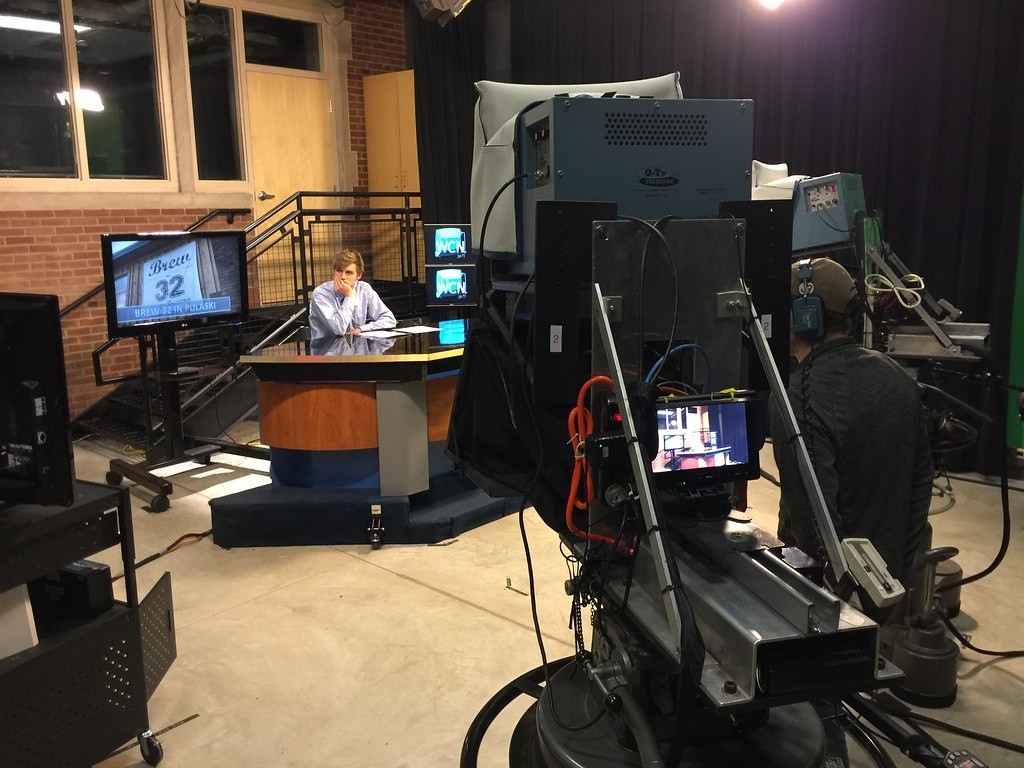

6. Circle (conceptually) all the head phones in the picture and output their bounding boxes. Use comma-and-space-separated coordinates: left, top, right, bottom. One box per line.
790, 258, 828, 340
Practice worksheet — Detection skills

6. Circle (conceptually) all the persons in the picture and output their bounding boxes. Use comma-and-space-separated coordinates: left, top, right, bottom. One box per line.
311, 336, 396, 358
307, 250, 396, 334
773, 257, 934, 611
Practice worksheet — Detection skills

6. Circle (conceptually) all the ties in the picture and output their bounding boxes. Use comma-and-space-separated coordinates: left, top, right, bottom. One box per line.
342, 298, 350, 333
344, 334, 351, 348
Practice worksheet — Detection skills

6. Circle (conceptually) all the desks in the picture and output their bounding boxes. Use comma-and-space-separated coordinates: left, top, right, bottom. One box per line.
676, 445, 731, 469
238, 318, 465, 496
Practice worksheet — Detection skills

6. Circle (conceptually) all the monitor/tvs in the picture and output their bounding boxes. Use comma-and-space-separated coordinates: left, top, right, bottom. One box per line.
424, 224, 478, 350
100, 231, 249, 339
0, 290, 75, 506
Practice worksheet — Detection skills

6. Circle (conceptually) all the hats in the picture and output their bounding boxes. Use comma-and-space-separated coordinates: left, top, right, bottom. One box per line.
791, 257, 858, 313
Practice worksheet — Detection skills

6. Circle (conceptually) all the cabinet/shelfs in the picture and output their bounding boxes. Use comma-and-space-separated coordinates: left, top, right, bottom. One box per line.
0, 479, 178, 767
